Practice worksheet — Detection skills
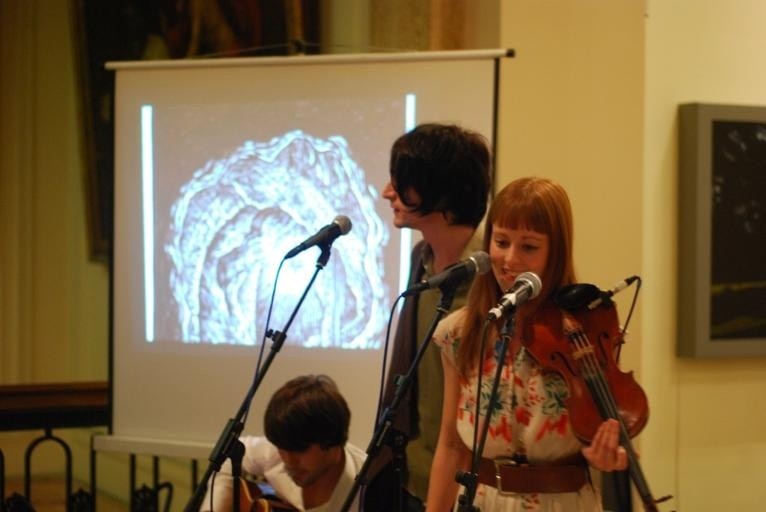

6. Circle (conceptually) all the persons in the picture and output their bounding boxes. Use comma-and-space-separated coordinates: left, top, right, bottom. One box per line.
198, 372, 371, 512
356, 122, 505, 508
425, 178, 641, 512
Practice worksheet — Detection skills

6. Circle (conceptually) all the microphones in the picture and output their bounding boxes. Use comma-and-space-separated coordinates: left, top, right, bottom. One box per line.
587, 275, 636, 310
487, 271, 542, 322
285, 215, 352, 258
401, 250, 492, 297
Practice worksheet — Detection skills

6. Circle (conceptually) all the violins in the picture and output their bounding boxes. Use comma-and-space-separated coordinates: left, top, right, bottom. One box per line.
520, 282, 673, 512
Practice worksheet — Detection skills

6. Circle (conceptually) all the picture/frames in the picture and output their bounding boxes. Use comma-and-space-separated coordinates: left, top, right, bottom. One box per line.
681, 101, 766, 357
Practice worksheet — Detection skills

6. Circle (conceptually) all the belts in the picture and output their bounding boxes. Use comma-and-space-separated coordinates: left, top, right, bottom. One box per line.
466, 451, 588, 496
